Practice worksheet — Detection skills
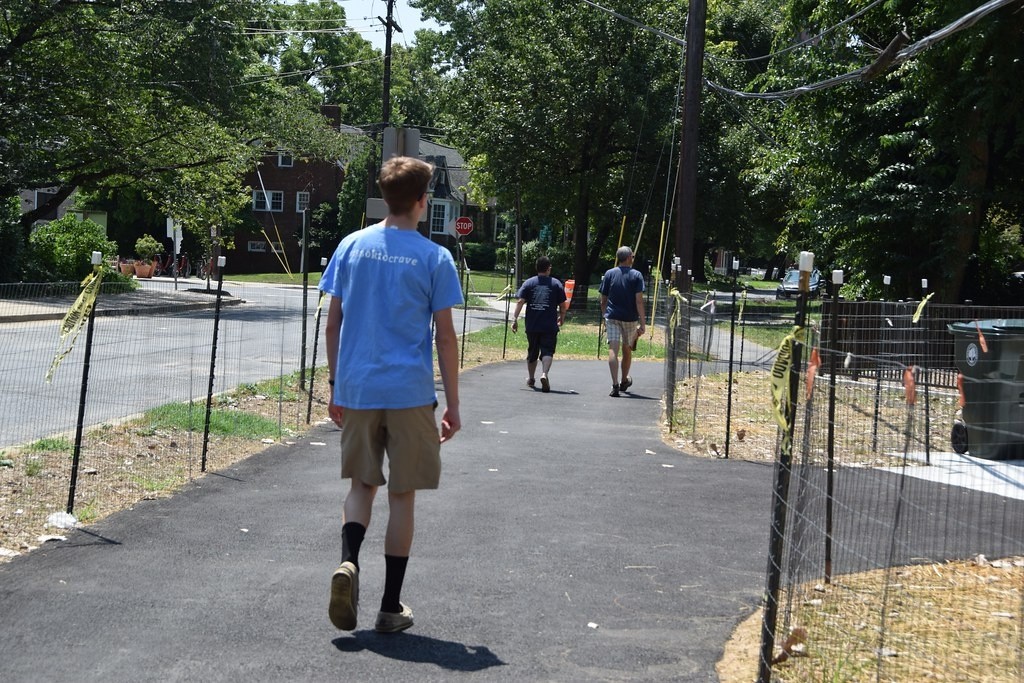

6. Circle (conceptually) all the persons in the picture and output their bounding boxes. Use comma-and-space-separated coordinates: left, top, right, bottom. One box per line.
598, 246, 647, 397
511, 256, 567, 392
318, 154, 466, 633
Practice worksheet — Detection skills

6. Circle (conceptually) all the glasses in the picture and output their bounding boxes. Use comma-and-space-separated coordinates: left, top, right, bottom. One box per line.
549, 265, 553, 269
629, 255, 635, 258
417, 188, 435, 202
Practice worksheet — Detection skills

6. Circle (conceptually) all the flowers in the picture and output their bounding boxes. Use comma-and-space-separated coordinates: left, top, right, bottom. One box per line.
121, 257, 148, 265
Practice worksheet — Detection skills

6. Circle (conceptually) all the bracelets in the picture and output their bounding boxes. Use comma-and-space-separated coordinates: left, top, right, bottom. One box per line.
329, 380, 335, 386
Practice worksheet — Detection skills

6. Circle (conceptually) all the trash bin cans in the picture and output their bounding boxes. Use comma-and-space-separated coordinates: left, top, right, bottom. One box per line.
947, 318, 1024, 460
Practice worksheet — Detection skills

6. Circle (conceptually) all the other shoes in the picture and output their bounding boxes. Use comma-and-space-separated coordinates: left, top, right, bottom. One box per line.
609, 390, 620, 397
540, 377, 550, 392
619, 375, 633, 391
526, 378, 535, 387
375, 601, 414, 633
328, 561, 360, 632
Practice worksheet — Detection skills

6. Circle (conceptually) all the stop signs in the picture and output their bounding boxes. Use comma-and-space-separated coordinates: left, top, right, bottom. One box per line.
455, 217, 473, 235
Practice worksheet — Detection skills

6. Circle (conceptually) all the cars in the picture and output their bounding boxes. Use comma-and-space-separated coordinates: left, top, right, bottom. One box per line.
776, 267, 828, 301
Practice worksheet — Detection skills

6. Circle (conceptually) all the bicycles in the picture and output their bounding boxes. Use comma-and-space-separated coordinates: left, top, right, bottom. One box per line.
171, 253, 192, 277
154, 253, 173, 277
197, 253, 213, 280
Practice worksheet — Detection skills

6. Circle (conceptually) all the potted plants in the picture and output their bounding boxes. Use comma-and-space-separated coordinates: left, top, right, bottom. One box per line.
134, 234, 164, 278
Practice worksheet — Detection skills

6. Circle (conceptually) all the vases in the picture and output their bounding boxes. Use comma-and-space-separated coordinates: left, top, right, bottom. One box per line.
120, 264, 134, 275
134, 265, 151, 278
